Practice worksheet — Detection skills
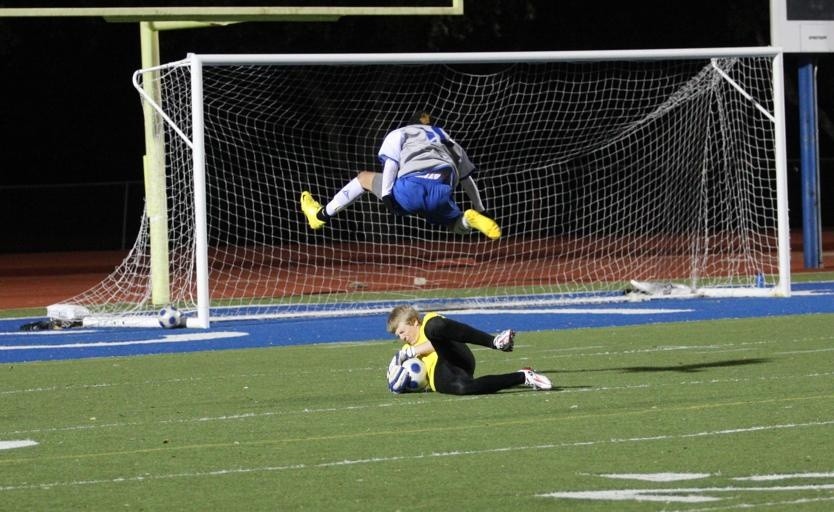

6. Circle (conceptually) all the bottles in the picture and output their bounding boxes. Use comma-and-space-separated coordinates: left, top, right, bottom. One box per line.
756, 273, 763, 288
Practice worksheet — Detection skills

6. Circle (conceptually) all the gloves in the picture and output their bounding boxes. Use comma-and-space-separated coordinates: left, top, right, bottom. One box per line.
480, 211, 493, 219
386, 346, 416, 394
383, 195, 403, 217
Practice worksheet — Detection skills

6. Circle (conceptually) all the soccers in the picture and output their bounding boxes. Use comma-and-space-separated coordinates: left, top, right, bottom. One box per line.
388, 354, 429, 393
158, 307, 182, 328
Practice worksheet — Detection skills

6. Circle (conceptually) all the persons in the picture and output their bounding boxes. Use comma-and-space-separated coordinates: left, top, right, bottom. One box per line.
384, 304, 553, 396
298, 106, 503, 243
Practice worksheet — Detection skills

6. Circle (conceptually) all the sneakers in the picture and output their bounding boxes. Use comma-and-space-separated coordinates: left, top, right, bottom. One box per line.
299, 190, 327, 231
517, 367, 552, 391
463, 208, 502, 240
493, 329, 515, 353
